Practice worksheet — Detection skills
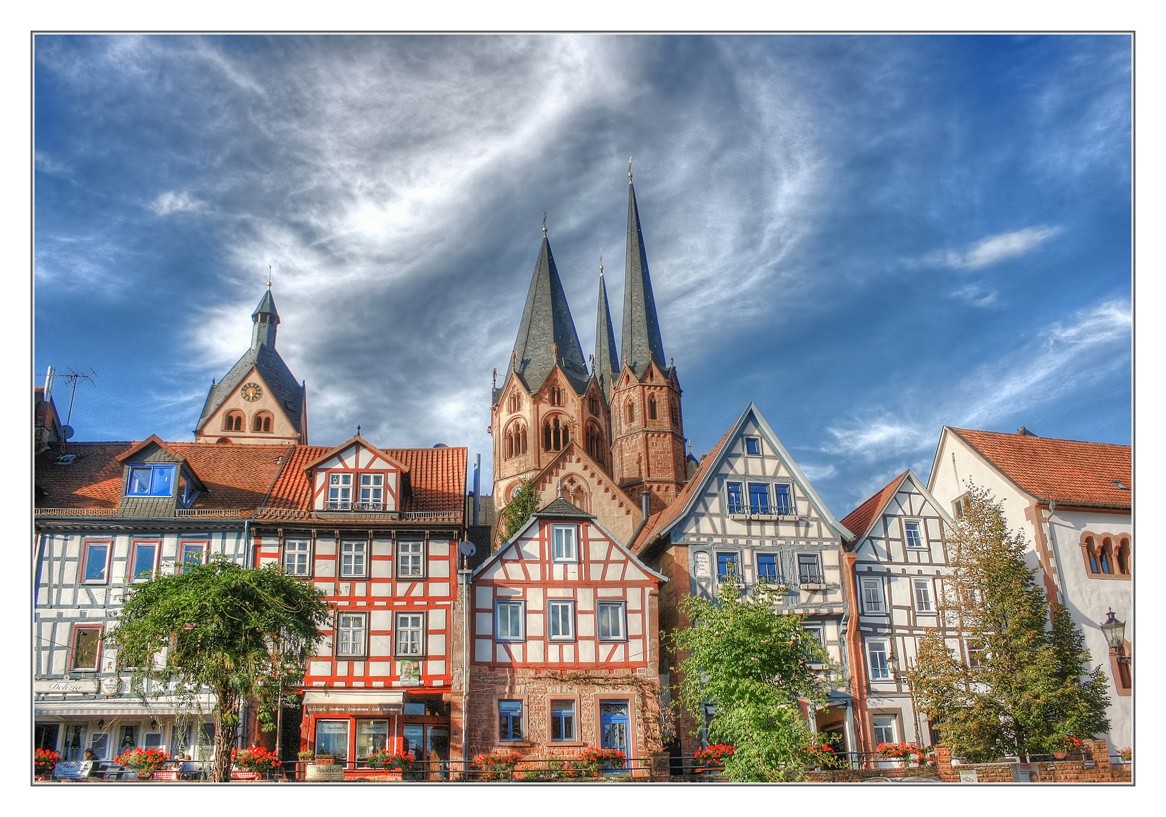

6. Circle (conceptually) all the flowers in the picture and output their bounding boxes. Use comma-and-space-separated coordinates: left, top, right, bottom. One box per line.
692, 744, 735, 773
231, 746, 282, 772
113, 747, 169, 777
805, 743, 835, 764
579, 748, 626, 776
368, 750, 415, 768
876, 742, 933, 767
471, 752, 522, 778
34, 748, 59, 772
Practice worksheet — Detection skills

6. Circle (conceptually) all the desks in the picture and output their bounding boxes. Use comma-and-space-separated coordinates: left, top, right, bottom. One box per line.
153, 768, 181, 779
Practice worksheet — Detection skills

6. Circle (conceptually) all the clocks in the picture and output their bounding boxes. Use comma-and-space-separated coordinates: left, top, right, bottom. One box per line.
240, 383, 263, 402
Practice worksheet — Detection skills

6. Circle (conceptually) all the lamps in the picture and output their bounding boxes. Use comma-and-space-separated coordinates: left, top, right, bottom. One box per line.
98, 720, 104, 730
150, 720, 156, 730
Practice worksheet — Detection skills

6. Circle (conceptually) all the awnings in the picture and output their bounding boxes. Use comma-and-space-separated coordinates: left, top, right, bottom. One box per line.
302, 692, 404, 714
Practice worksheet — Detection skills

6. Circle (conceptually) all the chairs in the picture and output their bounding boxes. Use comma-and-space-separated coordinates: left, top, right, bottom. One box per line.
96, 765, 109, 779
108, 767, 125, 780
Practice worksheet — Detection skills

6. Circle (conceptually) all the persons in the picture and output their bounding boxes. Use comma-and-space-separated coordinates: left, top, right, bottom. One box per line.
400, 662, 420, 686
84, 747, 100, 777
49, 751, 69, 782
116, 734, 135, 779
174, 753, 197, 780
